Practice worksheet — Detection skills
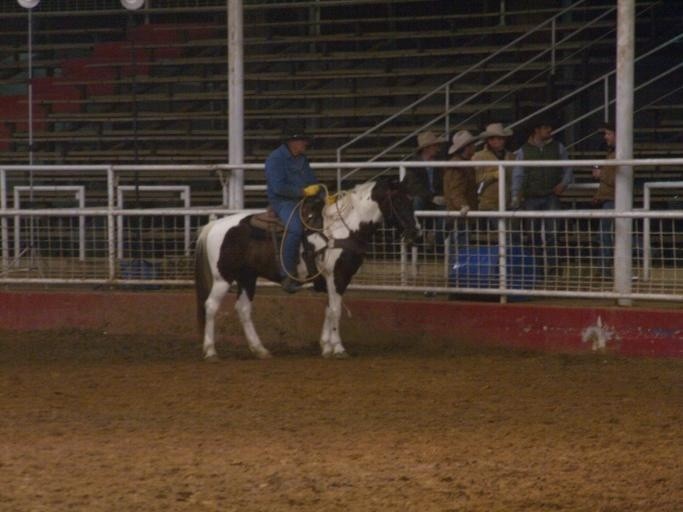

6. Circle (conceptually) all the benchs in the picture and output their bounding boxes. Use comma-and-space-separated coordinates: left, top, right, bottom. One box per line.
0, 0, 682, 262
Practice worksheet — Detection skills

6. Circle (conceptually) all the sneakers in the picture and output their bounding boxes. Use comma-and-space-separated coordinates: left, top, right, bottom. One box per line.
281, 278, 299, 294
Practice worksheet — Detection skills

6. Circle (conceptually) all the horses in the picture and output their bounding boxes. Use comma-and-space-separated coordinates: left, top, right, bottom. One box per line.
193, 173, 426, 365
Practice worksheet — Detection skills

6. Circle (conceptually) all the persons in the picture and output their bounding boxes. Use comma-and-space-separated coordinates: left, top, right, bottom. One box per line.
441, 128, 480, 284
510, 115, 574, 276
590, 120, 638, 283
399, 130, 445, 281
470, 122, 519, 244
262, 122, 336, 294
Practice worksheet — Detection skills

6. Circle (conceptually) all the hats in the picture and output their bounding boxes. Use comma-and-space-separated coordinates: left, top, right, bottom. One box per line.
523, 113, 556, 134
447, 128, 477, 156
477, 121, 513, 139
602, 120, 615, 132
413, 131, 445, 153
274, 120, 312, 142
